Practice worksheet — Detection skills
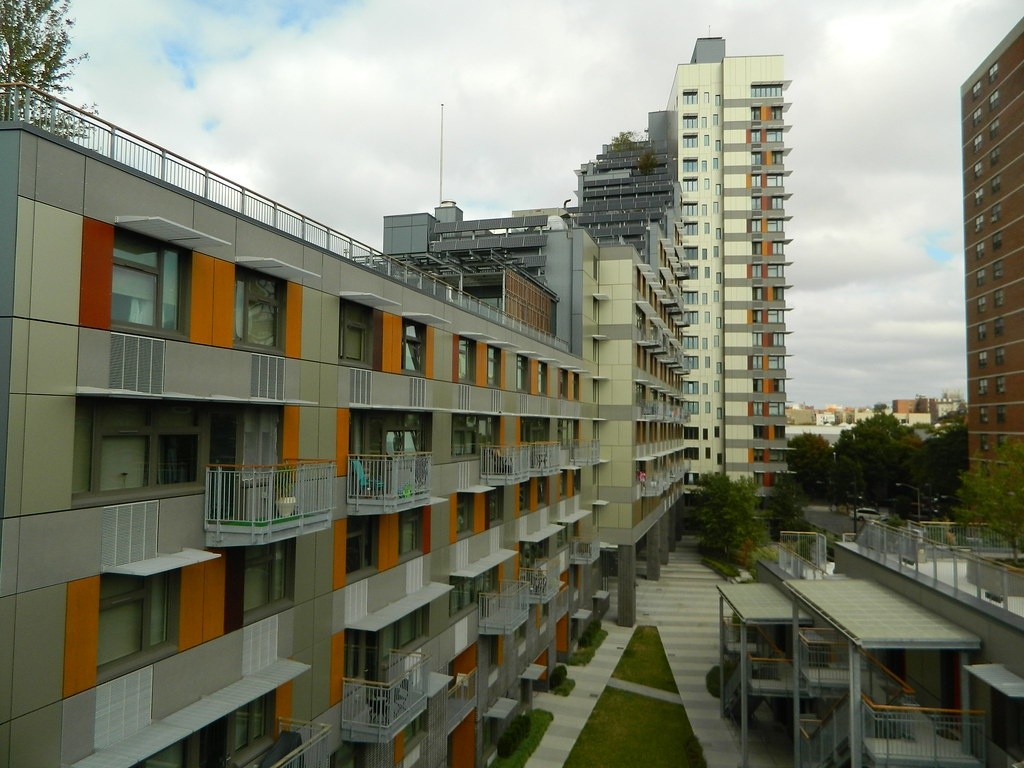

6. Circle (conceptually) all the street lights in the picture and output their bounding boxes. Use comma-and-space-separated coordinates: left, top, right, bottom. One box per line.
895, 482, 920, 524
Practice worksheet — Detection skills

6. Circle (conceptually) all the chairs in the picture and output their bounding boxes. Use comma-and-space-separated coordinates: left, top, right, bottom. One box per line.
353, 460, 384, 496
369, 678, 410, 724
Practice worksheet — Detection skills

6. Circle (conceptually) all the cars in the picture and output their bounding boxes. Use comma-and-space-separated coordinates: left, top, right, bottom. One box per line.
849, 507, 889, 522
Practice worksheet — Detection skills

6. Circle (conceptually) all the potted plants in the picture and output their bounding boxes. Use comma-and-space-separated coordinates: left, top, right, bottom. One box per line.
275, 465, 297, 516
